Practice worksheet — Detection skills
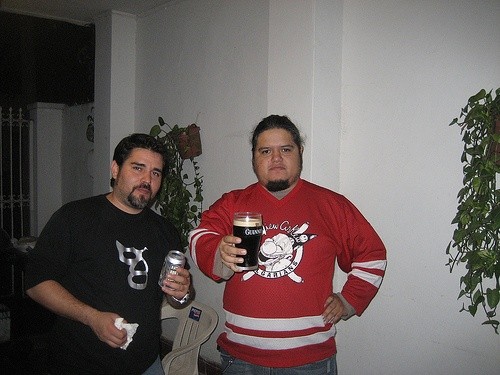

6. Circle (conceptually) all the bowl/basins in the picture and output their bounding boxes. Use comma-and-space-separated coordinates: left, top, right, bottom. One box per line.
18, 239, 37, 252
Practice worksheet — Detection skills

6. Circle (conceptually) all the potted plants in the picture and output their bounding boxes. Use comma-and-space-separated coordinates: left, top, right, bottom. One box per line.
444, 87, 500, 336
148, 116, 204, 254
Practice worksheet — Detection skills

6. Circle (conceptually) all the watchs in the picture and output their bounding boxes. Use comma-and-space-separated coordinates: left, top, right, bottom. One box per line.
171, 291, 191, 305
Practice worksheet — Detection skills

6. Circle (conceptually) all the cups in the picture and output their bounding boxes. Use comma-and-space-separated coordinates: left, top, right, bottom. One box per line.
233, 212, 262, 270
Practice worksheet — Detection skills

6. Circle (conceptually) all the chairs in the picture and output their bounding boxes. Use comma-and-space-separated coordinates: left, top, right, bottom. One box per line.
160, 300, 219, 375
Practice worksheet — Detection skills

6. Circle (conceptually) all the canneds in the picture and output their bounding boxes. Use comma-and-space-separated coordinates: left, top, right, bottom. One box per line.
158, 250, 186, 291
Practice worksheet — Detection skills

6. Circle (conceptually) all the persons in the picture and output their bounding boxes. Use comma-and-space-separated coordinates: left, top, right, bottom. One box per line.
188, 115, 388, 375
22, 133, 197, 375
